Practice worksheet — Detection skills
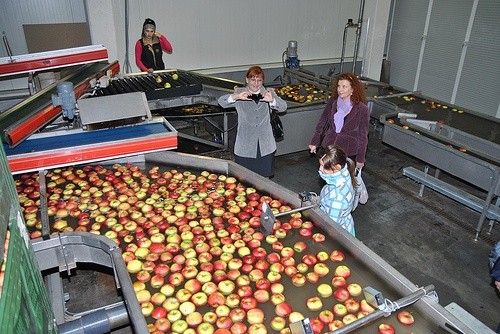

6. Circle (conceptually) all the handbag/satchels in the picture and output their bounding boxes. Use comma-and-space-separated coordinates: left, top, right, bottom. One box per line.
268, 91, 285, 138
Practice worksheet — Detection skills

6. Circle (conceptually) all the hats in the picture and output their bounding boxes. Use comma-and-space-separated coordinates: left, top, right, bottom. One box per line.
143, 18, 156, 31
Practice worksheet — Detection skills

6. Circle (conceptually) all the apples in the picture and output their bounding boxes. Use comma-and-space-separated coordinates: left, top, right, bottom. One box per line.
378, 323, 395, 334
397, 310, 414, 326
403, 125, 409, 130
387, 119, 395, 124
164, 82, 171, 88
397, 94, 464, 113
172, 74, 178, 80
274, 84, 332, 103
437, 119, 445, 124
0, 165, 377, 334
388, 91, 393, 95
459, 147, 466, 152
156, 77, 162, 83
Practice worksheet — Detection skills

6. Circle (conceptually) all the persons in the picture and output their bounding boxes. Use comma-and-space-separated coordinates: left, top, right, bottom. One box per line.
489, 240, 500, 291
309, 73, 369, 190
218, 66, 287, 180
312, 145, 369, 237
135, 18, 173, 73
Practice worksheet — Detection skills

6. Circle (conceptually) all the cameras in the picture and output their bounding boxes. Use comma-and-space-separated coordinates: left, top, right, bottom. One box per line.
247, 93, 264, 100
